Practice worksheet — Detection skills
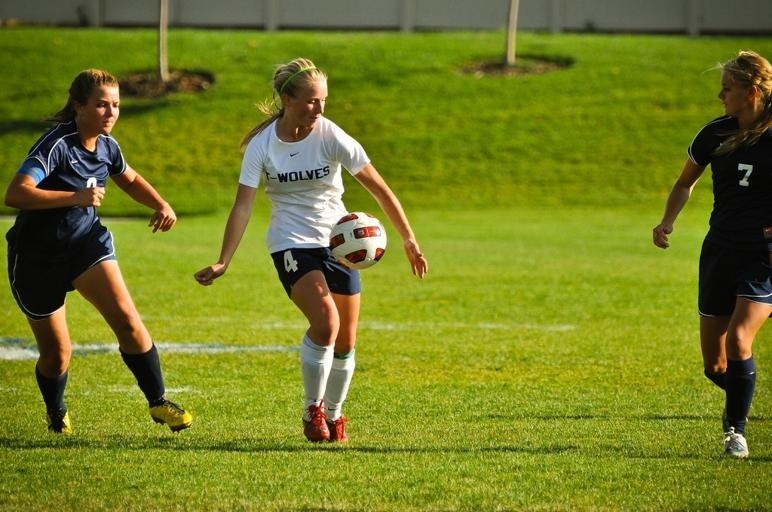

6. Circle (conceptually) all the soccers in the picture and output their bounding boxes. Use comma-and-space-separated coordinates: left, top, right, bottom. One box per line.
331, 212, 387, 269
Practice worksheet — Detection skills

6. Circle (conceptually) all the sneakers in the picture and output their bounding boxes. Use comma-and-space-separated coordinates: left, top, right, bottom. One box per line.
47, 406, 73, 441
722, 409, 750, 458
302, 403, 331, 443
326, 413, 349, 444
149, 397, 195, 433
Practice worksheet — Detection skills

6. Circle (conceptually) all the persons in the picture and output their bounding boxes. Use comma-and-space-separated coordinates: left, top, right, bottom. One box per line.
194, 56, 428, 442
653, 50, 771, 459
5, 68, 192, 434
